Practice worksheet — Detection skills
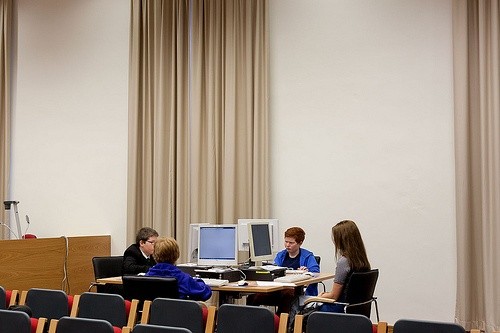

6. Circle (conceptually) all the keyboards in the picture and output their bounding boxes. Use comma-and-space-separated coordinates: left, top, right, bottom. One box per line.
286, 270, 307, 273
199, 278, 229, 287
274, 273, 311, 283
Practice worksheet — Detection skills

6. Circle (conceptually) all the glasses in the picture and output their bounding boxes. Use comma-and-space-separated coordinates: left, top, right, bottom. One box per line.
145, 240, 155, 245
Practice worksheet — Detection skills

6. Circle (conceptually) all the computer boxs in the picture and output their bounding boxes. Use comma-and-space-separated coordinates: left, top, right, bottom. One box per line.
177, 266, 287, 282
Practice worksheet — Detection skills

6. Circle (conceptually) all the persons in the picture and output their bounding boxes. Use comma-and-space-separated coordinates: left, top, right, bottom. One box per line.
122, 227, 159, 276
145, 236, 212, 302
298, 220, 371, 315
246, 226, 320, 317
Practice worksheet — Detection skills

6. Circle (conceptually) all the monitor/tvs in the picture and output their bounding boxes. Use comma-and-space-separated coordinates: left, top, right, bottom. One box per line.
187, 219, 280, 272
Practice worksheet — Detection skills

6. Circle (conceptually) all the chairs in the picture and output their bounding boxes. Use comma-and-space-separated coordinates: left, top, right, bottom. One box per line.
0, 257, 485, 333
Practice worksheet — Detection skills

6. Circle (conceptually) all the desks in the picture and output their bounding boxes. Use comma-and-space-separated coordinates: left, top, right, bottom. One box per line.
96, 267, 336, 310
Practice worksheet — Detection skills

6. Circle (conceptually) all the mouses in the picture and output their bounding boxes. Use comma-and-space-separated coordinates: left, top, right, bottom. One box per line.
304, 272, 315, 277
238, 281, 248, 286
